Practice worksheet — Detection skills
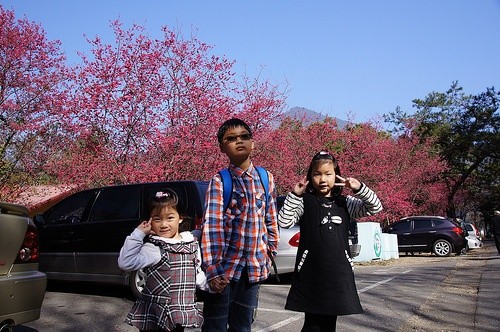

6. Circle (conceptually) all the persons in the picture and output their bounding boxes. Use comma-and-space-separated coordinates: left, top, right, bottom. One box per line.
455, 216, 466, 256
277, 151, 383, 332
201, 118, 280, 332
118, 188, 227, 332
489, 210, 500, 256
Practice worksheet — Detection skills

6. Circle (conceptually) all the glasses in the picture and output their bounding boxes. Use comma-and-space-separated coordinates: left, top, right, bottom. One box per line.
222, 133, 252, 141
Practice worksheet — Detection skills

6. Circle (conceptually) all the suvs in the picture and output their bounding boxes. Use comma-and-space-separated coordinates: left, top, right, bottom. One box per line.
34, 179, 210, 300
381, 215, 469, 257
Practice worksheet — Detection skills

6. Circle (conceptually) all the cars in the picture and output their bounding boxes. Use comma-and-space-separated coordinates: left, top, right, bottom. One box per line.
459, 222, 483, 254
267, 195, 359, 278
0, 199, 49, 332
